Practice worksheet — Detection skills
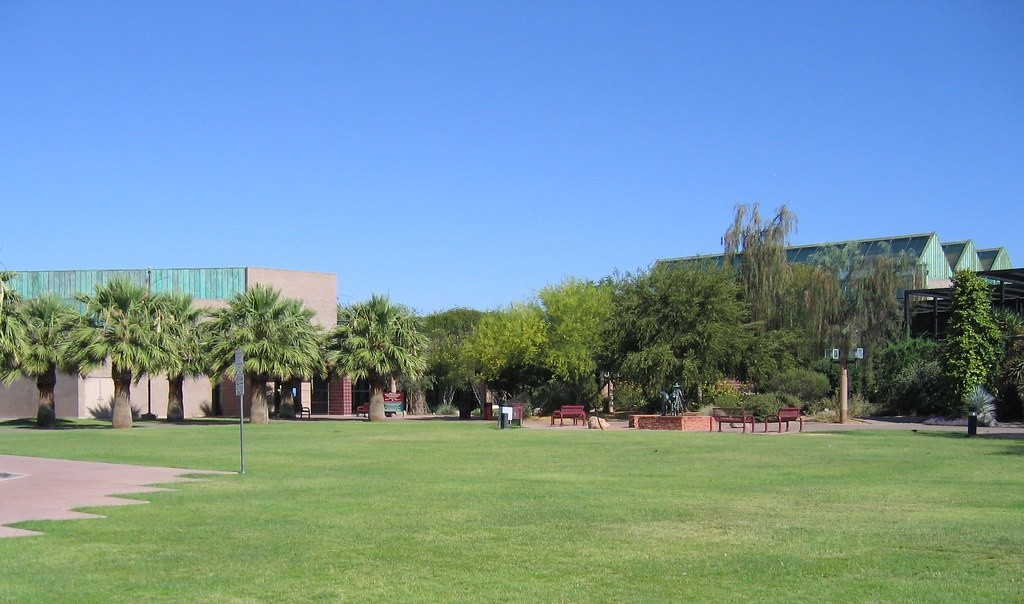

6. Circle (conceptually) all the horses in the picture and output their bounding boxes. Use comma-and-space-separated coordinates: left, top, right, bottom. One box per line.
659, 391, 683, 416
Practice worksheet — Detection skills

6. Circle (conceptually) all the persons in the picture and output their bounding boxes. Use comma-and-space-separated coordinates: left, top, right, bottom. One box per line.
658, 383, 685, 416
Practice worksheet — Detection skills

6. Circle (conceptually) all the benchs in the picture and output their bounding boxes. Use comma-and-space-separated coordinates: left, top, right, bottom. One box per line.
765, 407, 802, 433
551, 406, 586, 427
709, 408, 754, 433
356, 403, 369, 417
294, 403, 310, 419
